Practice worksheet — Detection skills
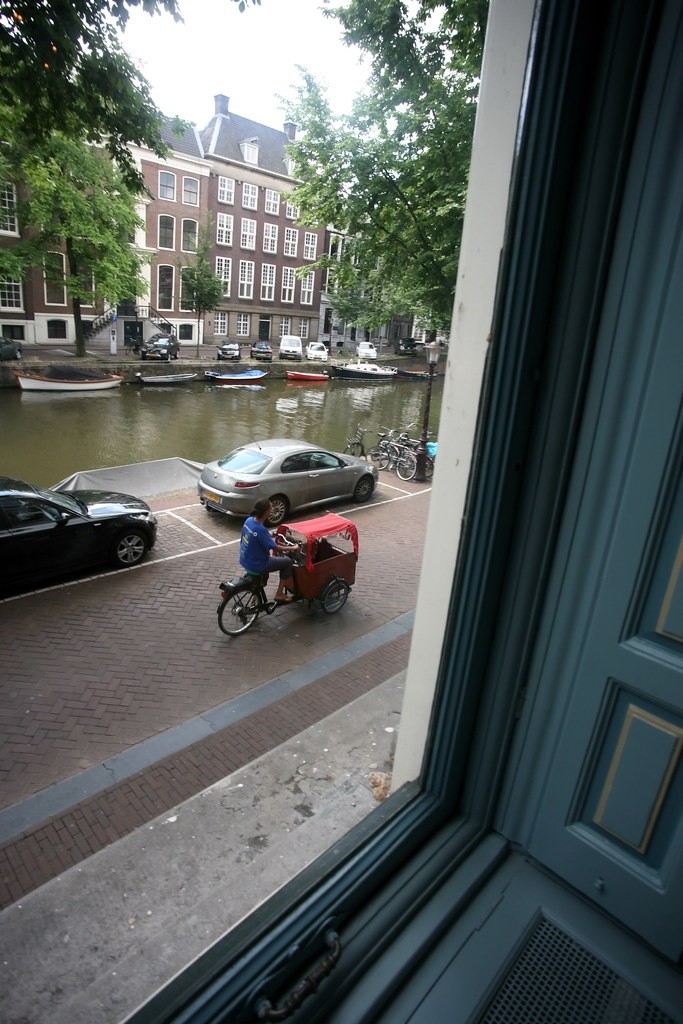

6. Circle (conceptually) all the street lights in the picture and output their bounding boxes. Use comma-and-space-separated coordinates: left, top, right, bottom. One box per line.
411, 339, 444, 483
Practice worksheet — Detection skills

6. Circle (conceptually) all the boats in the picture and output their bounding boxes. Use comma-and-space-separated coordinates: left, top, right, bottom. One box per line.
394, 367, 438, 378
206, 369, 269, 383
285, 369, 329, 381
331, 363, 399, 379
9, 367, 125, 392
133, 372, 199, 385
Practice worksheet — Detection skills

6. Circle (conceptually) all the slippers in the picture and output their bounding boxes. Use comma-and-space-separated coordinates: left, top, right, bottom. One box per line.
274, 592, 293, 602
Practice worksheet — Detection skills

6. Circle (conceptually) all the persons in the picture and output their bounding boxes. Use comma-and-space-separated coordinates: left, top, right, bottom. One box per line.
239, 496, 295, 602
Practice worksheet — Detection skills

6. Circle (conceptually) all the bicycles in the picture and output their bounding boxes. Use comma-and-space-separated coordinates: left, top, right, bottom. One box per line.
338, 421, 435, 481
125, 337, 140, 355
214, 528, 354, 637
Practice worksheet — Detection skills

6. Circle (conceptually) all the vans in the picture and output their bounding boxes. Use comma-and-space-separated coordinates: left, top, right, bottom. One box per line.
278, 335, 303, 361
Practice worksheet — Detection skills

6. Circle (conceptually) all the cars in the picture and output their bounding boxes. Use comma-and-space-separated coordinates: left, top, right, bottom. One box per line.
196, 432, 380, 526
249, 342, 272, 363
355, 341, 377, 360
0, 473, 157, 600
140, 333, 181, 362
305, 342, 330, 361
216, 340, 242, 363
